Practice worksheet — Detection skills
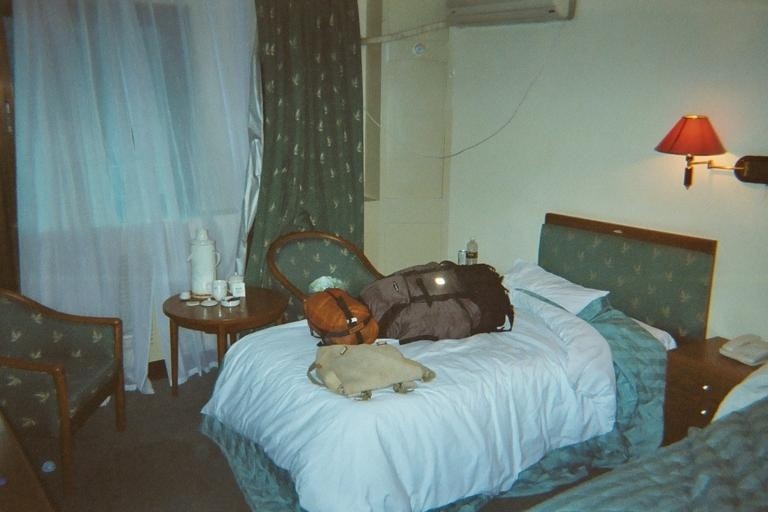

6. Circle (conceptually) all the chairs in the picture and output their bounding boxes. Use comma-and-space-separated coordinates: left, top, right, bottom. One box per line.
262, 229, 385, 322
0, 286, 127, 499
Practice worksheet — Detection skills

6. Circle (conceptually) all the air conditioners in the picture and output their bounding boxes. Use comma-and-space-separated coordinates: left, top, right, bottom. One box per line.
446, 0, 570, 28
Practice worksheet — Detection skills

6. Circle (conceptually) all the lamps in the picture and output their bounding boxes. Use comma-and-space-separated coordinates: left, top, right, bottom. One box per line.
654, 114, 768, 191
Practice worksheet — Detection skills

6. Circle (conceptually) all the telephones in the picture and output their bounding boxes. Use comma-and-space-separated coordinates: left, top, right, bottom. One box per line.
718, 334, 768, 366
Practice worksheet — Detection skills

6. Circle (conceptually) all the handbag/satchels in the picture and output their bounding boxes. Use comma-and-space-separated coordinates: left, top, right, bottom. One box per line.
359, 261, 514, 344
304, 288, 379, 344
308, 340, 434, 400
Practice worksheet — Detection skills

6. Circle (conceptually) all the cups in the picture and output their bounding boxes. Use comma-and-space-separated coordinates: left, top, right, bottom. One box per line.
213, 280, 227, 302
226, 272, 244, 295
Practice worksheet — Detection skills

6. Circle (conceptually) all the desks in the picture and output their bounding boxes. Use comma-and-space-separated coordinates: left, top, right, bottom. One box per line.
162, 286, 289, 397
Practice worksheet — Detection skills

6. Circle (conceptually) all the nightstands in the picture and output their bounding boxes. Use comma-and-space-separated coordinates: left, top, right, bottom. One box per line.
661, 334, 766, 442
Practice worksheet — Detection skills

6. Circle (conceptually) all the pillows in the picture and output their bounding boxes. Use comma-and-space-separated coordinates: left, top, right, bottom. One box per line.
500, 259, 610, 313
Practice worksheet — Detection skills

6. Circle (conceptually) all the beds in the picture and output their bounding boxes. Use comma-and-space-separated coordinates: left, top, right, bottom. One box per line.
199, 213, 717, 511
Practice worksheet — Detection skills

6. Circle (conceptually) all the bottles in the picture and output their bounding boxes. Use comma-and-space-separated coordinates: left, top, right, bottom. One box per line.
466, 237, 478, 266
186, 228, 221, 300
